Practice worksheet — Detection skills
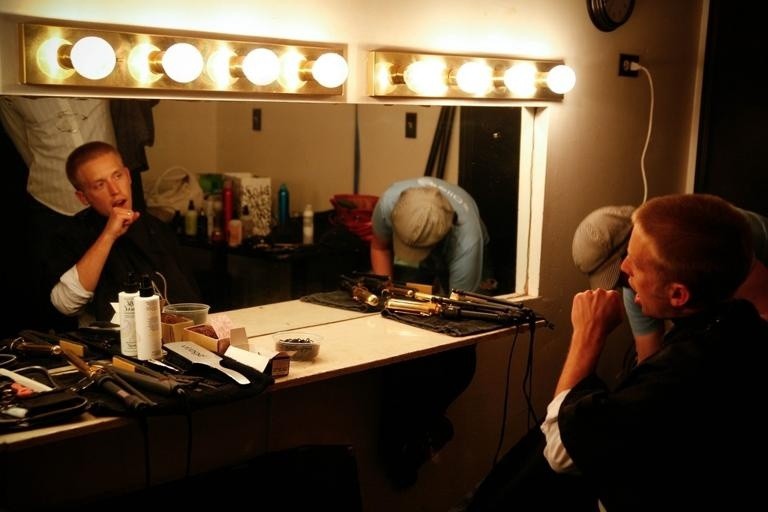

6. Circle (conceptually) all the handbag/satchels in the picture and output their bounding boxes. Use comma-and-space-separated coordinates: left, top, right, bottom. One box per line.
145, 165, 204, 224
330, 194, 377, 241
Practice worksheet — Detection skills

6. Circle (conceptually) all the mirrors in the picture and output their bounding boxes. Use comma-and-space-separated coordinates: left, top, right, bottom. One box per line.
0, 92, 525, 368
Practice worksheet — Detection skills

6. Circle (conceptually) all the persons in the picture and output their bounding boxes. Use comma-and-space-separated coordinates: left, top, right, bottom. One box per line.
535, 190, 767, 512
0, 97, 129, 336
570, 203, 766, 367
25, 134, 203, 337
363, 174, 486, 299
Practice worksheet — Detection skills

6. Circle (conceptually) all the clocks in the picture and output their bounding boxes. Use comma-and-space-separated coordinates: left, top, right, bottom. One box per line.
587, 0, 635, 33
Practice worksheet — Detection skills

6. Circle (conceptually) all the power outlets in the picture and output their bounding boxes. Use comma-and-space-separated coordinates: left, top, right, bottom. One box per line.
619, 53, 640, 78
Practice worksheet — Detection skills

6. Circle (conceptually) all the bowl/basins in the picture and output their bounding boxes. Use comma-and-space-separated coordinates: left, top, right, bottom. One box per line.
273, 331, 321, 367
162, 302, 210, 323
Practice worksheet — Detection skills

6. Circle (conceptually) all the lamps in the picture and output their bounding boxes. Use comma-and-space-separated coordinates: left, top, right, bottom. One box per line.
376, 59, 441, 92
437, 61, 494, 95
532, 66, 575, 95
127, 43, 204, 82
278, 51, 347, 90
486, 62, 538, 96
34, 36, 116, 81
206, 45, 279, 87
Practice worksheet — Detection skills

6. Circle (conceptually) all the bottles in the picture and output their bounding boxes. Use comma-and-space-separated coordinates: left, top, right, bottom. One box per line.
241, 204, 253, 241
185, 199, 196, 237
223, 178, 233, 242
301, 203, 314, 244
196, 208, 207, 242
228, 209, 242, 247
211, 217, 223, 244
278, 183, 290, 222
205, 194, 217, 240
175, 210, 183, 235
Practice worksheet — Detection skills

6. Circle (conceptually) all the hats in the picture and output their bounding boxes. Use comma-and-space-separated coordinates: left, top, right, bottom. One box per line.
571, 206, 637, 291
391, 186, 454, 264
